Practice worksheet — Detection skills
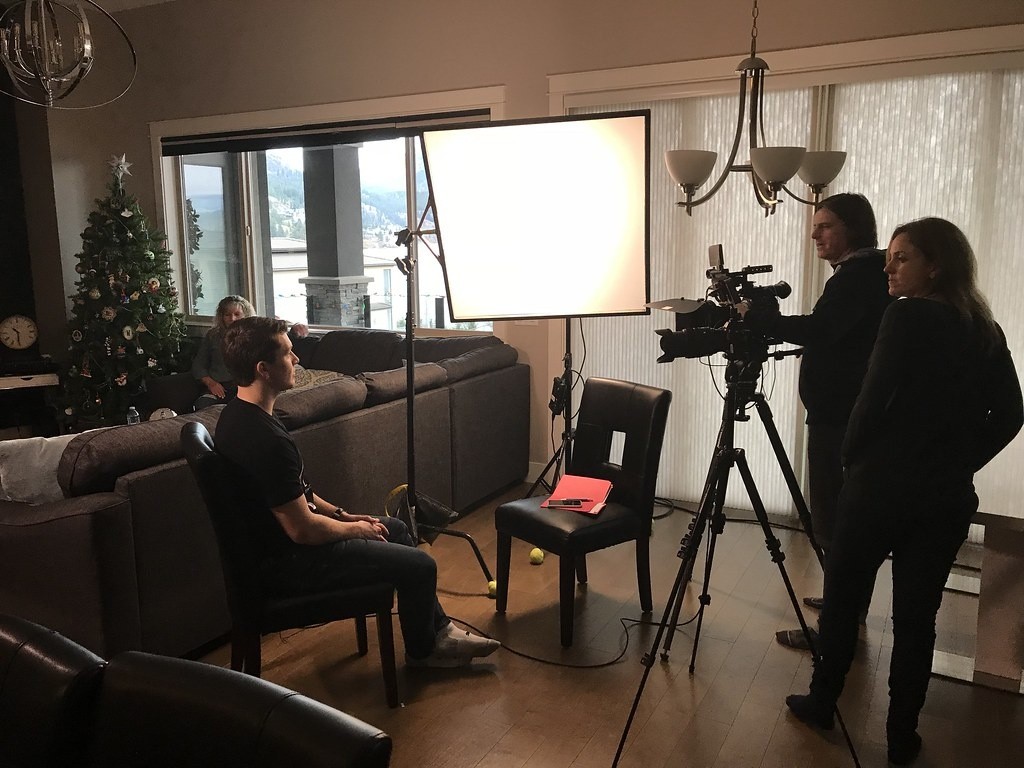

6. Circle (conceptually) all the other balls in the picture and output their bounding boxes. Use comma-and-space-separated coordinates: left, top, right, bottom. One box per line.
530, 548, 544, 564
488, 580, 497, 596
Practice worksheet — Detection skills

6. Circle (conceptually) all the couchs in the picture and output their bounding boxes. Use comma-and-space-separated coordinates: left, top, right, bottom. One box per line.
0, 328, 530, 657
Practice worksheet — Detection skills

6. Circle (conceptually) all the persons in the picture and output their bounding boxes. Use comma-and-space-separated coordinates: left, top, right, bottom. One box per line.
784, 218, 1024, 768
209, 316, 501, 669
169, 296, 308, 413
734, 193, 896, 651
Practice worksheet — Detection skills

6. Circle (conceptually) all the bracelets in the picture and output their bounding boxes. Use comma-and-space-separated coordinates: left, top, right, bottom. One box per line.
332, 508, 342, 518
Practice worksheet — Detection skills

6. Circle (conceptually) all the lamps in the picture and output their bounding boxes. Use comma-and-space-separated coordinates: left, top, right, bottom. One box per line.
666, 0, 847, 219
0, 0, 138, 111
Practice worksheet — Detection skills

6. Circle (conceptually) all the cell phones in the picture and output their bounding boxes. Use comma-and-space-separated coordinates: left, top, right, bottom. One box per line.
549, 499, 583, 508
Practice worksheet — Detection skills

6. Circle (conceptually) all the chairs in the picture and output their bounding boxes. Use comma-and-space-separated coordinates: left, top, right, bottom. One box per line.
493, 376, 672, 651
181, 421, 403, 713
0, 612, 105, 768
107, 650, 392, 768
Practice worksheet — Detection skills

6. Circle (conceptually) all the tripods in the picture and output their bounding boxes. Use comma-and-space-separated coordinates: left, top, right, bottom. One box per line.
613, 347, 862, 768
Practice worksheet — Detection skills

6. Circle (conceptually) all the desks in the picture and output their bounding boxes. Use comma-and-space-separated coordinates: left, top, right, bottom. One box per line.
0, 361, 60, 417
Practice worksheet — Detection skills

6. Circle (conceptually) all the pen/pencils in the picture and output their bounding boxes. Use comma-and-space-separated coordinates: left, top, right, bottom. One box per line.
561, 498, 593, 502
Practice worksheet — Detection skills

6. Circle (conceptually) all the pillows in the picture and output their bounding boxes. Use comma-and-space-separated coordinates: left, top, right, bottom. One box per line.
0, 431, 78, 504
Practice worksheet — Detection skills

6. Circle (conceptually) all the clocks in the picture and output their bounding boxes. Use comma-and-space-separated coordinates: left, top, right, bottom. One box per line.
1, 318, 35, 350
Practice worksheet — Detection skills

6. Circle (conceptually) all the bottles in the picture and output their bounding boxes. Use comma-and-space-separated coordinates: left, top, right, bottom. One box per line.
127, 406, 140, 425
